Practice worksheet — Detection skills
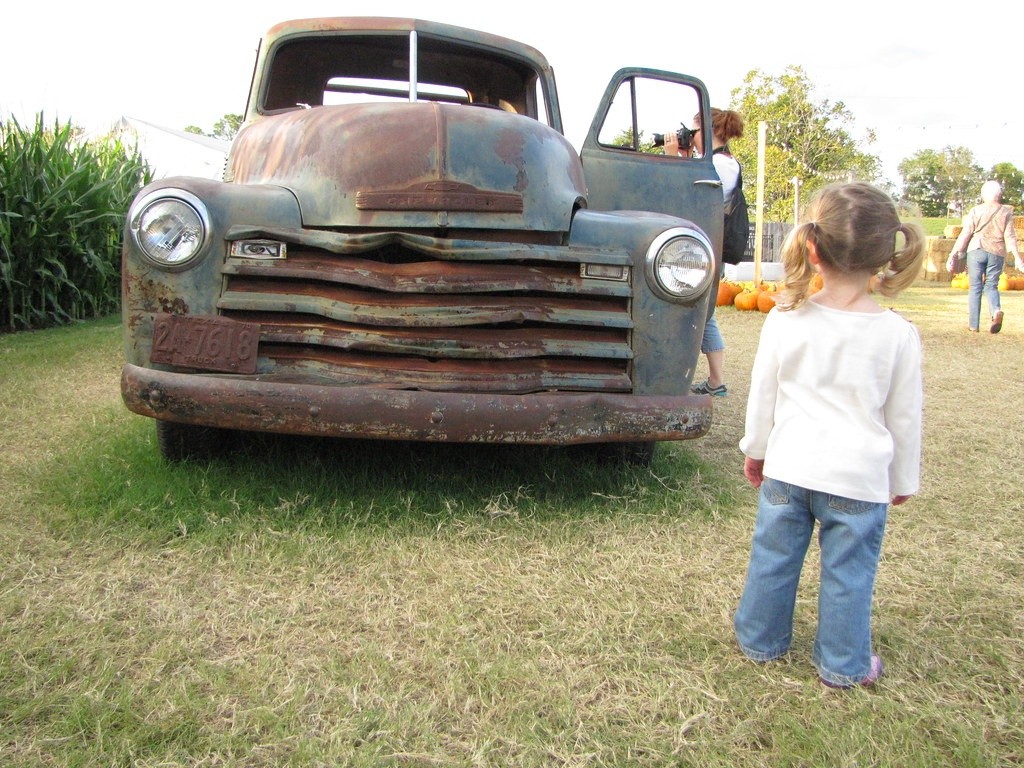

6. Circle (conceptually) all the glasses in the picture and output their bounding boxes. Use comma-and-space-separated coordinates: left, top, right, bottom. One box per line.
691, 129, 701, 137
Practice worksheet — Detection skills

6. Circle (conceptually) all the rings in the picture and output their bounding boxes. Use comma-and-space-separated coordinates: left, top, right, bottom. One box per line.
667, 140, 670, 143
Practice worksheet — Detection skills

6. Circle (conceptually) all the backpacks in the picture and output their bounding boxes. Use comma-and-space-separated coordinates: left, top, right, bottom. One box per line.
722, 160, 749, 266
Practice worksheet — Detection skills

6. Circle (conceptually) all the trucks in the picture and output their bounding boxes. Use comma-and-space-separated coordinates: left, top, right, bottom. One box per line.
120, 15, 725, 470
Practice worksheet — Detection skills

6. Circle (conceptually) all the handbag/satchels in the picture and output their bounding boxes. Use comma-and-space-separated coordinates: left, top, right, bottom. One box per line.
950, 252, 967, 274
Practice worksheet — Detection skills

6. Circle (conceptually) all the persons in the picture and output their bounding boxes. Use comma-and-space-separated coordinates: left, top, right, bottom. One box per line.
664, 108, 742, 398
945, 180, 1024, 334
736, 181, 924, 687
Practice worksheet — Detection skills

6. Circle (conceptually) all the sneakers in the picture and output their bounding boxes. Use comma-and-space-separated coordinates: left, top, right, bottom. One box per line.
690, 376, 727, 397
820, 654, 882, 692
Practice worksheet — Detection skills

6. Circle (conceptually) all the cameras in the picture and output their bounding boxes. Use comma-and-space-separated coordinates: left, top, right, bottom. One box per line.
651, 123, 693, 149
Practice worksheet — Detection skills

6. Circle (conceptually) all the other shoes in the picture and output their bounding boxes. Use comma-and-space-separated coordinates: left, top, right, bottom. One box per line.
969, 328, 978, 333
990, 311, 1003, 334
